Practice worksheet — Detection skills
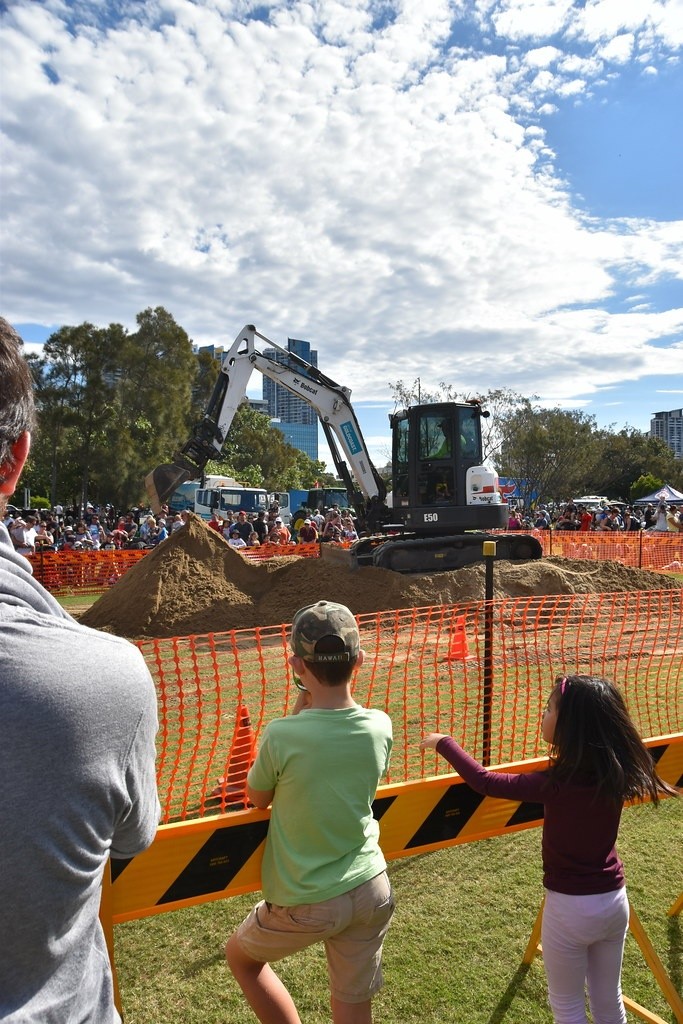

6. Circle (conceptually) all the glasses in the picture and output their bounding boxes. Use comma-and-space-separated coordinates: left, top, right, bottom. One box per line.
248, 518, 253, 520
92, 516, 98, 520
276, 522, 281, 524
40, 525, 46, 528
304, 523, 309, 526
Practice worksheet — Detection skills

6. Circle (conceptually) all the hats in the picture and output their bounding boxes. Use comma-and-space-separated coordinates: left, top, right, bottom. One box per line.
15, 520, 27, 528
107, 533, 114, 537
118, 516, 126, 522
158, 519, 166, 525
604, 506, 609, 510
290, 600, 360, 662
64, 526, 73, 531
539, 510, 546, 516
239, 511, 246, 515
232, 530, 239, 533
275, 517, 282, 522
304, 519, 312, 525
314, 508, 319, 512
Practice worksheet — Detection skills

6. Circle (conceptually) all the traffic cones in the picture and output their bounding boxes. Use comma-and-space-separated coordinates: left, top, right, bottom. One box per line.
208, 702, 263, 804
442, 614, 478, 661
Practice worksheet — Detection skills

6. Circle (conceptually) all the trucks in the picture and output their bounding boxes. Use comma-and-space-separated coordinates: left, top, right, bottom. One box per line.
299, 486, 357, 527
166, 475, 270, 537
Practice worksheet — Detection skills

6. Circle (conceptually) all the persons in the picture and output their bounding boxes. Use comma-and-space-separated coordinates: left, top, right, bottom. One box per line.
419, 675, 683, 1024
506, 496, 683, 569
0, 316, 161, 1024
2, 498, 358, 591
420, 418, 466, 504
226, 600, 395, 1024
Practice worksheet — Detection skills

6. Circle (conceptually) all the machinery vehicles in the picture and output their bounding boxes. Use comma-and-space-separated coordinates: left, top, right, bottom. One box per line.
145, 323, 542, 574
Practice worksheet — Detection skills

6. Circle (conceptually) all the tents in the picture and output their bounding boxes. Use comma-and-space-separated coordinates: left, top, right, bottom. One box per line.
634, 484, 683, 506
